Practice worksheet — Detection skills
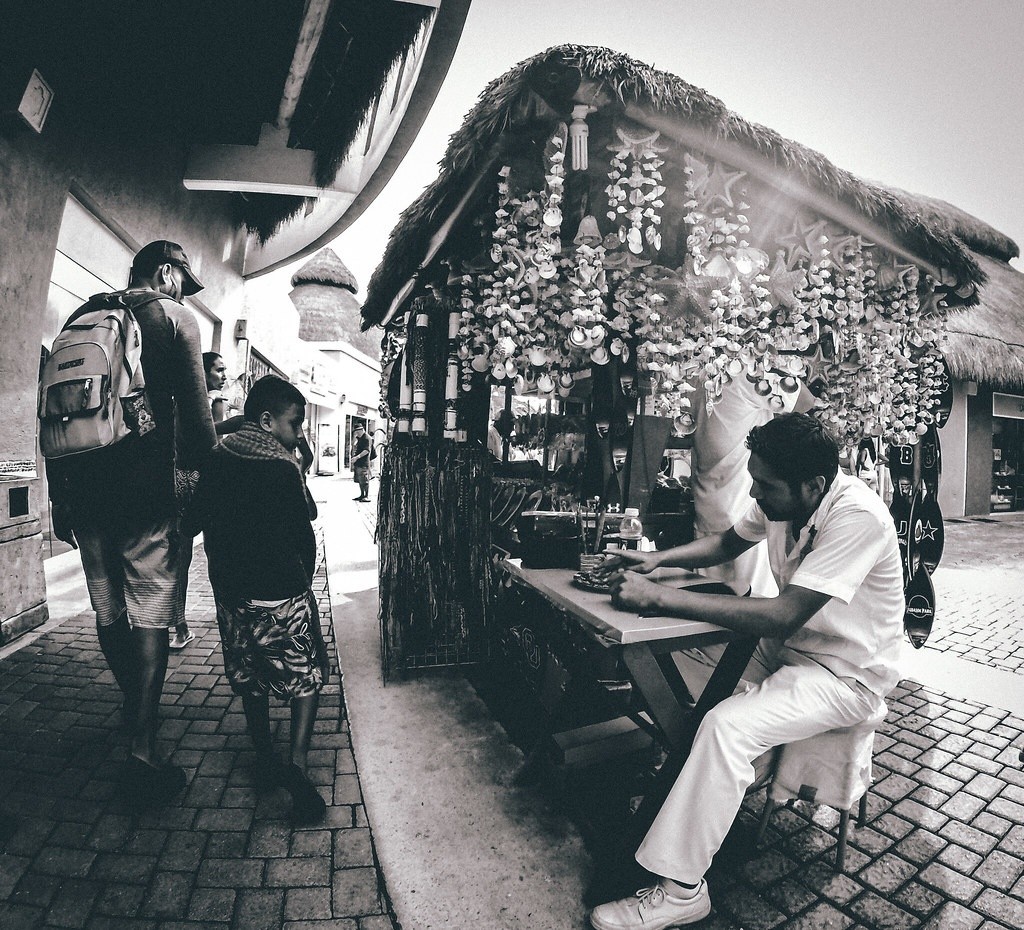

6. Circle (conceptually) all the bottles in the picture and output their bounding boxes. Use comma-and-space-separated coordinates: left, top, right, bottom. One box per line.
619, 508, 643, 551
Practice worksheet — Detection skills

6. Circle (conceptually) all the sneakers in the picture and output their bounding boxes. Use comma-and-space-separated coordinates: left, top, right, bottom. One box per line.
745, 746, 774, 794
590, 876, 711, 930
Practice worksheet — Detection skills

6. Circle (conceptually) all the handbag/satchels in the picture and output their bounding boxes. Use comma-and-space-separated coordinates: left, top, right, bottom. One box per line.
369, 445, 377, 460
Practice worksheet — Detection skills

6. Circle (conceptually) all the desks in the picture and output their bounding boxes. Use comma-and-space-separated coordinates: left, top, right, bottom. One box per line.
498, 555, 772, 882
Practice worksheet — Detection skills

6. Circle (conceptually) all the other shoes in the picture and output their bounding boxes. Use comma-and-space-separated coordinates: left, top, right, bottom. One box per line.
359, 497, 372, 502
254, 741, 275, 777
119, 698, 144, 728
353, 496, 365, 500
131, 741, 176, 771
168, 622, 193, 648
280, 761, 325, 820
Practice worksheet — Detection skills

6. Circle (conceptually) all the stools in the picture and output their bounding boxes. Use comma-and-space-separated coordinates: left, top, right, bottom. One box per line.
753, 697, 888, 875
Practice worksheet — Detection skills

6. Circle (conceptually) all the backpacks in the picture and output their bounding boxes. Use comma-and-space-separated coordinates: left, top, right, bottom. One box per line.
39, 290, 183, 460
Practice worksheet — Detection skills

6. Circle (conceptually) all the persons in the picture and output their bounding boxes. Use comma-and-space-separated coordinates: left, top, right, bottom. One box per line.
588, 412, 909, 930
487, 408, 518, 463
35, 240, 331, 821
349, 423, 374, 502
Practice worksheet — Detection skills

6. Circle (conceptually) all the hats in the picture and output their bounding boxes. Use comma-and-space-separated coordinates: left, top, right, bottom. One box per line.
352, 423, 364, 432
131, 240, 205, 297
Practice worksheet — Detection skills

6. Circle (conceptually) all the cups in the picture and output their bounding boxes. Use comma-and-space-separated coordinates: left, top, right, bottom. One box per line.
580, 553, 604, 574
